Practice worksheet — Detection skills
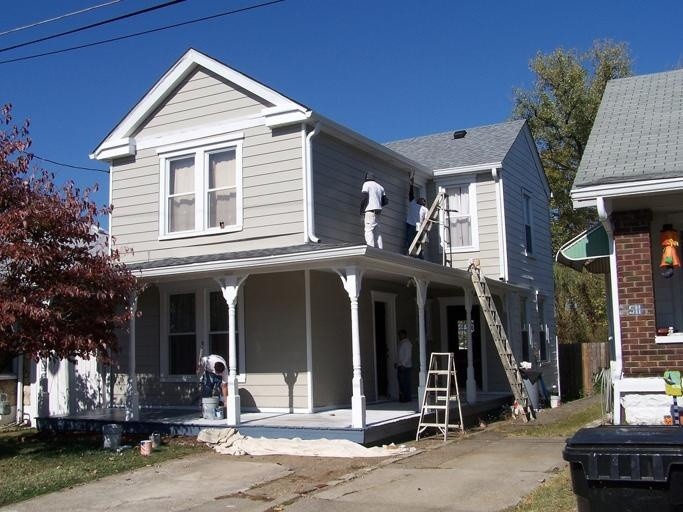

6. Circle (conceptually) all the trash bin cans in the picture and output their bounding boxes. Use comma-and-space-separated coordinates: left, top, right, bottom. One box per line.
562, 424, 683, 512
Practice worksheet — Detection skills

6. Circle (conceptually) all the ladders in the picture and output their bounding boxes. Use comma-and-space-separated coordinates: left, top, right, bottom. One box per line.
415, 352, 465, 443
467, 263, 536, 423
405, 192, 452, 268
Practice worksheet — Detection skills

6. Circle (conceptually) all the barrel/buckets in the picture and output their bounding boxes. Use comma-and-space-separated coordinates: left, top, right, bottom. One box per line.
101, 424, 123, 451
550, 396, 561, 408
201, 396, 220, 419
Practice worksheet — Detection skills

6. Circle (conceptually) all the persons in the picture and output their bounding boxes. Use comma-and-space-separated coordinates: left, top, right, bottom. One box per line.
358, 171, 389, 250
198, 353, 229, 410
394, 329, 413, 403
405, 169, 428, 259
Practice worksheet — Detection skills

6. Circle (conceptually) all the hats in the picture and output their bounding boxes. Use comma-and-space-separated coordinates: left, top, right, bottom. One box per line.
364, 172, 375, 181
